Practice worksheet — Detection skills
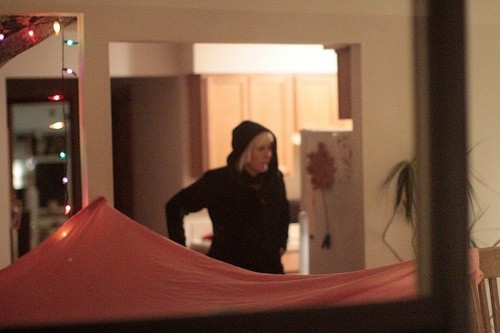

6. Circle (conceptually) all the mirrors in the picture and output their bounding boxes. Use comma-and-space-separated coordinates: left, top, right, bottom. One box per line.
3, 75, 83, 266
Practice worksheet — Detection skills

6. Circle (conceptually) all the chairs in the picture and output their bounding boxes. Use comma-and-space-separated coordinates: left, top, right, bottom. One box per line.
464, 247, 500, 332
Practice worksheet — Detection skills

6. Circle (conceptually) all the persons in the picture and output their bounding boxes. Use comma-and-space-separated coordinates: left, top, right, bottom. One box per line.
164, 120, 303, 275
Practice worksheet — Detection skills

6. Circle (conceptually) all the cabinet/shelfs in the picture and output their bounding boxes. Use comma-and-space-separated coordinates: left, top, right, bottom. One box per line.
190, 72, 352, 198
